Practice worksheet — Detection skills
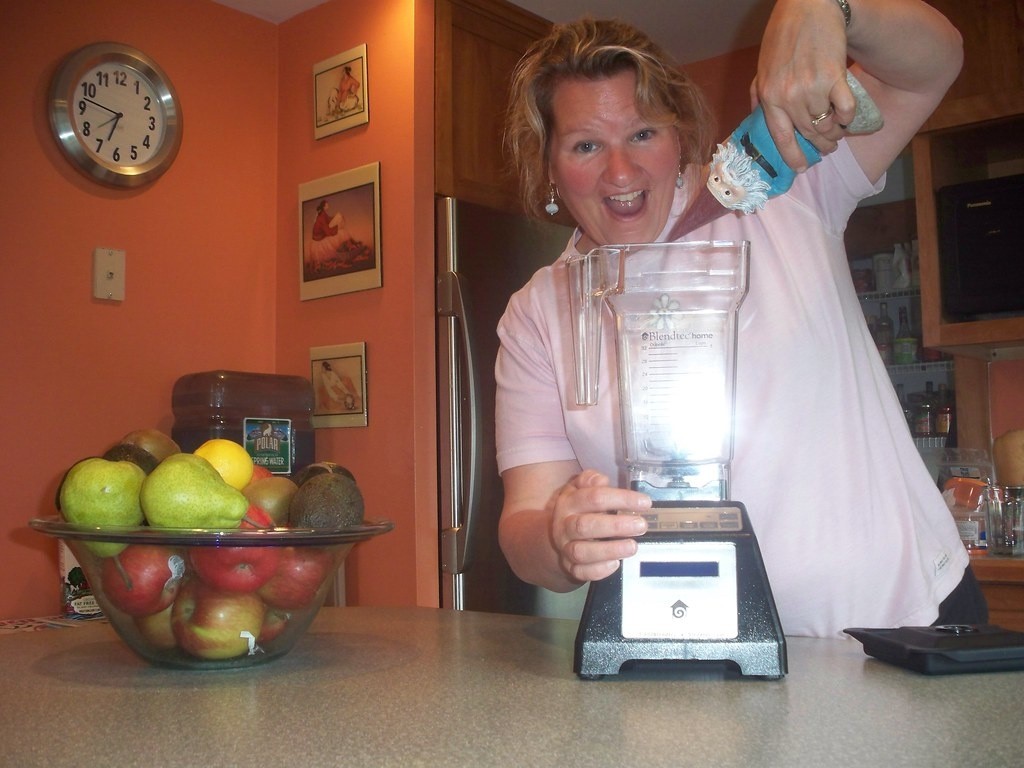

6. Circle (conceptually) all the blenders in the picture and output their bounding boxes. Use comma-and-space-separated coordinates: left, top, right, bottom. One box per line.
565, 239, 788, 680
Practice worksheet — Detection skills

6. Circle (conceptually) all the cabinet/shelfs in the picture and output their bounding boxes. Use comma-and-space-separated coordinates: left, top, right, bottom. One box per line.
911, 0, 1024, 358
431, 0, 578, 230
857, 286, 957, 447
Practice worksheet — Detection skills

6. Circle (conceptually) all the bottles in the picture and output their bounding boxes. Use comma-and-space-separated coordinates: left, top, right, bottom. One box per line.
874, 302, 893, 366
896, 381, 952, 438
893, 306, 918, 365
171, 370, 316, 479
982, 485, 1024, 557
894, 240, 920, 289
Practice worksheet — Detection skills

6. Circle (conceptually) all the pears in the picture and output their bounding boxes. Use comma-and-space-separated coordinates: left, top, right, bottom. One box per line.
58, 454, 249, 557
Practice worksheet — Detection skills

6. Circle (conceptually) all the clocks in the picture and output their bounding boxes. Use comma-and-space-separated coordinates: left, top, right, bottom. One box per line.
44, 41, 183, 191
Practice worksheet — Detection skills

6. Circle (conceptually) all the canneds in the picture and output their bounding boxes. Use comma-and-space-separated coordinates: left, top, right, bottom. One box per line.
902, 404, 952, 436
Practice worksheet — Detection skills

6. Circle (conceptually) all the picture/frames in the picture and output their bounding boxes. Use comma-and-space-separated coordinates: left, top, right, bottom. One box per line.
311, 43, 370, 141
295, 161, 386, 300
309, 342, 368, 430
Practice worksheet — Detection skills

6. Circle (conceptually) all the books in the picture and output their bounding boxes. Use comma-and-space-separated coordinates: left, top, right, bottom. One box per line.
57, 536, 106, 621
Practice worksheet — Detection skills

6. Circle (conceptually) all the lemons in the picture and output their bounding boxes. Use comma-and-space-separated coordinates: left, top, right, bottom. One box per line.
195, 440, 254, 490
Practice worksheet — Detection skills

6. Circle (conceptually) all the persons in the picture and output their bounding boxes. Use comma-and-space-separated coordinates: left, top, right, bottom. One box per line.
494, 0, 987, 638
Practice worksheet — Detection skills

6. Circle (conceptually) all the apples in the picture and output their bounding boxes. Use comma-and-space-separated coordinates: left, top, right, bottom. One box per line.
249, 463, 271, 480
99, 504, 335, 660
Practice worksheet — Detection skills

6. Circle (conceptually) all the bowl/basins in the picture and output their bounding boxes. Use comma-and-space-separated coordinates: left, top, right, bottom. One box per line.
30, 514, 394, 669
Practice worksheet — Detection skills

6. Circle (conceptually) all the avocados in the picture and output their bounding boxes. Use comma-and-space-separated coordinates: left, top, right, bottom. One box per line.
290, 461, 364, 527
104, 444, 159, 474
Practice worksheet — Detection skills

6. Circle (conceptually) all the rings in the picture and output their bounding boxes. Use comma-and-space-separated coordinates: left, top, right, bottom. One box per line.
812, 104, 833, 127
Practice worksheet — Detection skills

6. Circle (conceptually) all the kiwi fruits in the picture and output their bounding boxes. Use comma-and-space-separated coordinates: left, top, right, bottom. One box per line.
120, 429, 179, 463
242, 477, 299, 523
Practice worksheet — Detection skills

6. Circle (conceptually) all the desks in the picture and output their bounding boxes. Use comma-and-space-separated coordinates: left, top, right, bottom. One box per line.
0, 602, 1024, 768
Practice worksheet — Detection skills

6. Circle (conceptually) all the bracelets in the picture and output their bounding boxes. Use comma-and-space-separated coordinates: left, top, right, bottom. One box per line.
836, 0, 852, 26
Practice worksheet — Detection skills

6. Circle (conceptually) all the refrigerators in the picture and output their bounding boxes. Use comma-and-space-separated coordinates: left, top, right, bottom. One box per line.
436, 196, 590, 622
